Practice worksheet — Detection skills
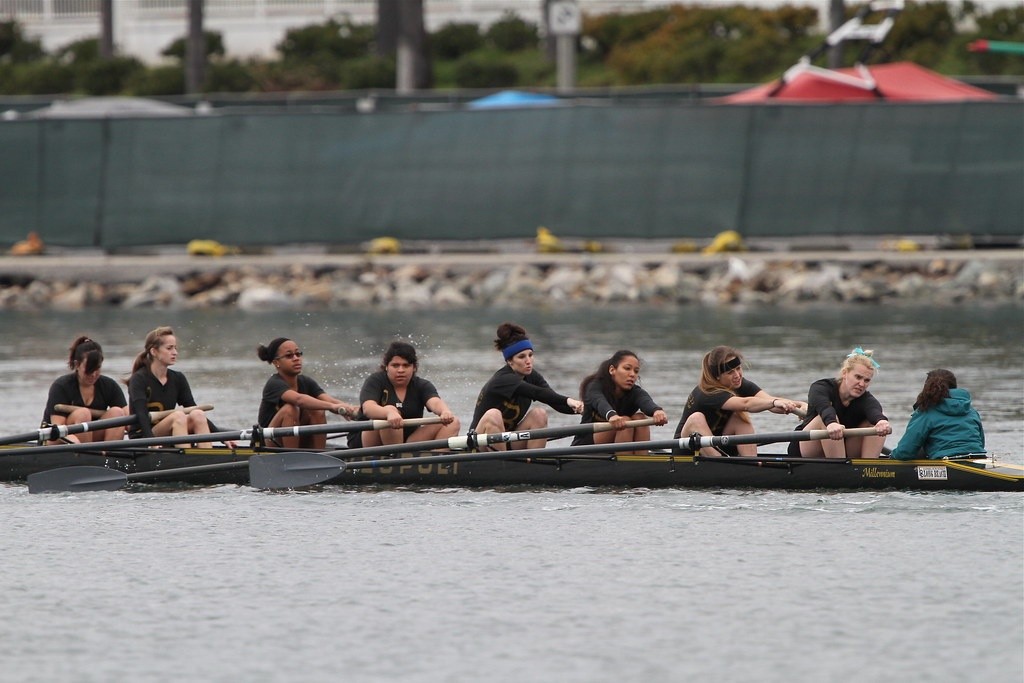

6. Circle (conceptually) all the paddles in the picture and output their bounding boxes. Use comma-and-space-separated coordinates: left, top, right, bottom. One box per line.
28, 414, 659, 496
249, 426, 892, 492
2, 404, 453, 454
788, 404, 891, 457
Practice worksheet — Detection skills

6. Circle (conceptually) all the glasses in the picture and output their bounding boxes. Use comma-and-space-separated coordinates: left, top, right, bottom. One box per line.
274, 351, 302, 360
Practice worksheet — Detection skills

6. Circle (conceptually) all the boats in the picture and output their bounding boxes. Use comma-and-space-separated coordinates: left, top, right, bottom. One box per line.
0, 401, 1024, 492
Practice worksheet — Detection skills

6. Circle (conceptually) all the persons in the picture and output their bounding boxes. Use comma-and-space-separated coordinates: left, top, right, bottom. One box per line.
787, 346, 892, 459
119, 327, 239, 450
258, 338, 361, 453
347, 341, 461, 452
889, 368, 988, 460
673, 346, 799, 457
468, 322, 585, 452
571, 350, 668, 456
39, 336, 130, 445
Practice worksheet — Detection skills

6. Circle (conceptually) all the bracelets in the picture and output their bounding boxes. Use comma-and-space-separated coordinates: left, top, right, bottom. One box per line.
772, 399, 777, 406
606, 409, 616, 421
